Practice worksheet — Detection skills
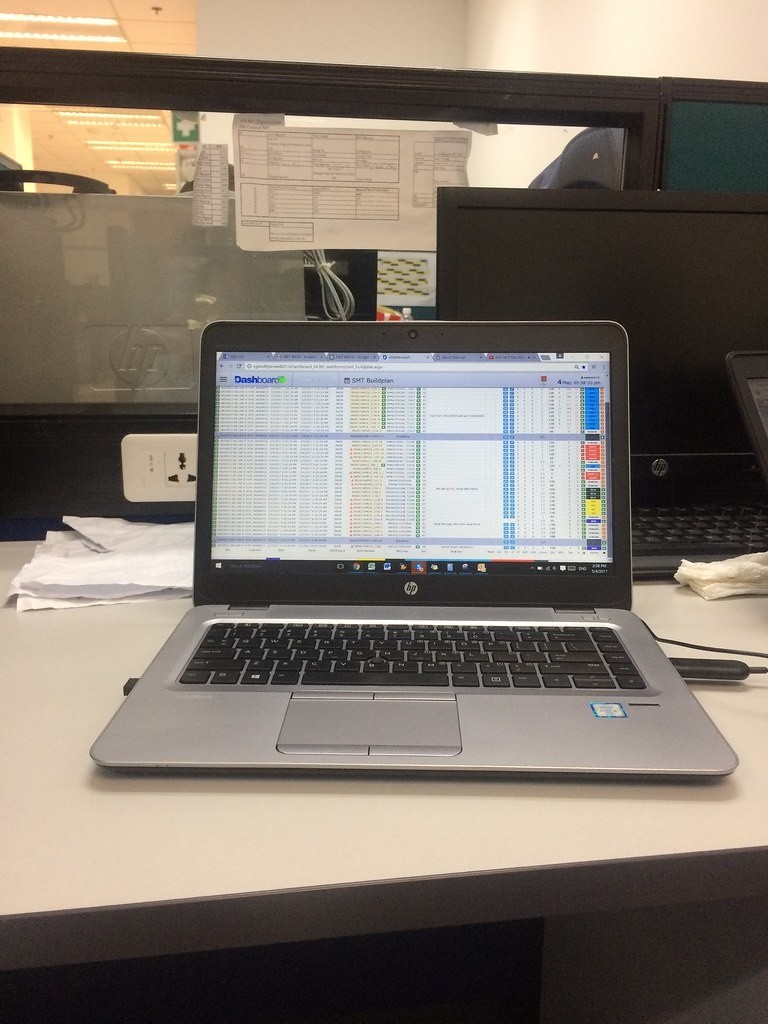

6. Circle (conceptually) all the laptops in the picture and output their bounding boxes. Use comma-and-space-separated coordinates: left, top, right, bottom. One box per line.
632, 501, 768, 580
89, 317, 740, 782
725, 349, 768, 489
0, 186, 306, 416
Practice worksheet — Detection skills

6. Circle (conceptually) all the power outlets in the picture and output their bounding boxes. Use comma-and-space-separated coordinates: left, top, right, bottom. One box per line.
119, 433, 196, 502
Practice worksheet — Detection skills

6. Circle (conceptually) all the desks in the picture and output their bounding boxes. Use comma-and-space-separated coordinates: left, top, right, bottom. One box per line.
0, 538, 768, 1024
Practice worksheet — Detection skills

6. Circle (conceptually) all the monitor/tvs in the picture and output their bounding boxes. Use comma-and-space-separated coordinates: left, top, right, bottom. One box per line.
436, 187, 768, 490
